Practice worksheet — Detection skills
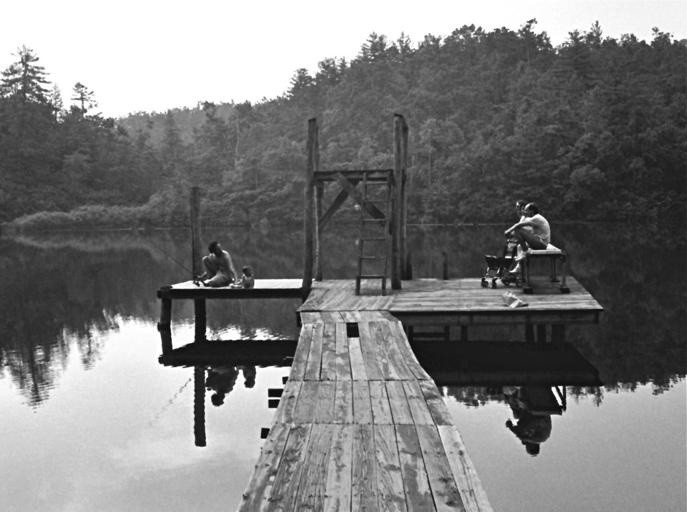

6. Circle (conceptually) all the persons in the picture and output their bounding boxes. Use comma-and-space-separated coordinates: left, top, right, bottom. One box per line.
504, 388, 552, 458
206, 366, 238, 406
198, 240, 238, 287
504, 200, 551, 273
231, 265, 255, 288
239, 363, 256, 388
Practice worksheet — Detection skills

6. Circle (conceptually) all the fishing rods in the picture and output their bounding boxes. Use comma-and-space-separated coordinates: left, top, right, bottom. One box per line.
130, 227, 204, 284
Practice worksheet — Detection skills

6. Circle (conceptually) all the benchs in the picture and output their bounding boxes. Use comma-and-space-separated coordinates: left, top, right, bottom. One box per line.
516, 241, 568, 294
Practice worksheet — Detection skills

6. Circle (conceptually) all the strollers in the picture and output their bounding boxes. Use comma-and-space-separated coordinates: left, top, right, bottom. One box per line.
480, 239, 523, 288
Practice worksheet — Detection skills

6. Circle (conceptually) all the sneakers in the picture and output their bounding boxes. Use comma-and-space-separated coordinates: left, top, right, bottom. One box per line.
514, 248, 532, 262
509, 267, 522, 274
